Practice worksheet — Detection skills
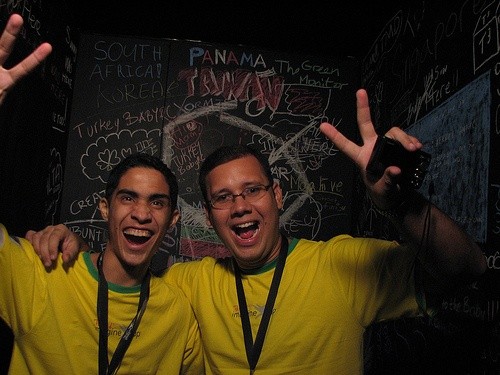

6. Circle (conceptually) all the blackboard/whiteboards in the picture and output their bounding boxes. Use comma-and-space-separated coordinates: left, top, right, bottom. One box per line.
0, 0, 500, 375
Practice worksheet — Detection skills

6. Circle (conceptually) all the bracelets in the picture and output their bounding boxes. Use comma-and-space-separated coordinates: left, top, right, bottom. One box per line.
375, 200, 408, 218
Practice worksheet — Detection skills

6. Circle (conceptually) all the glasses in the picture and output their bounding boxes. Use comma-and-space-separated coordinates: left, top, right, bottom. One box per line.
208, 181, 273, 208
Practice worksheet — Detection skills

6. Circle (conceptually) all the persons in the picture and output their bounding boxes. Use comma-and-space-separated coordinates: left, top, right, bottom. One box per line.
25, 88, 495, 374
0, 13, 205, 375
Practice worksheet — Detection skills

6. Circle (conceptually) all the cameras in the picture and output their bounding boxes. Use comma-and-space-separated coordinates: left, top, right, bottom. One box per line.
366, 136, 432, 189
